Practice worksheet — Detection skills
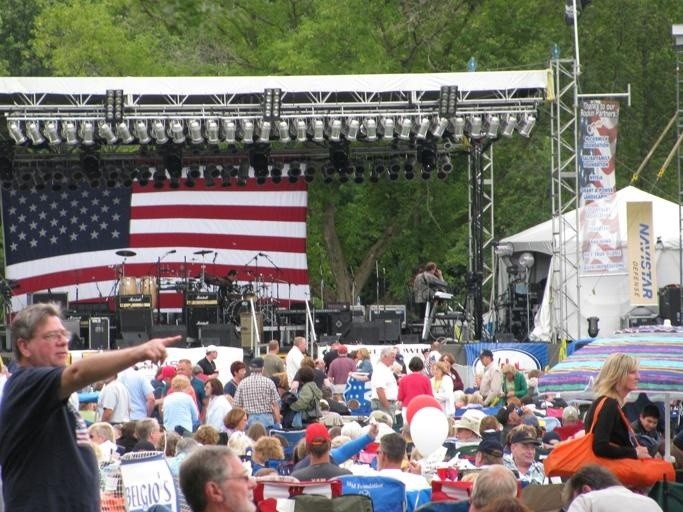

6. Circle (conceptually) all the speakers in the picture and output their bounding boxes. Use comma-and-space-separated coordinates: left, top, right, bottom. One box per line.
369, 305, 407, 329
114, 339, 145, 351
657, 284, 683, 325
339, 322, 378, 345
88, 317, 110, 351
66, 331, 82, 350
27, 291, 69, 319
343, 304, 366, 322
149, 324, 187, 347
326, 301, 352, 336
374, 319, 402, 341
116, 294, 153, 341
183, 291, 219, 347
201, 324, 241, 348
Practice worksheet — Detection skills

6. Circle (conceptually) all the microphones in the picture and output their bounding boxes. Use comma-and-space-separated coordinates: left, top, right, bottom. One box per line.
213, 252, 218, 262
259, 253, 266, 256
168, 250, 177, 253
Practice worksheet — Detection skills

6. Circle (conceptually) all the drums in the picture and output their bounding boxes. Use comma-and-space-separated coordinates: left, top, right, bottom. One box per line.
119, 272, 137, 295
138, 276, 157, 309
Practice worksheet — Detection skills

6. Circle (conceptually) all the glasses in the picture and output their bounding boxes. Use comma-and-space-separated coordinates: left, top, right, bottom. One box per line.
29, 330, 72, 342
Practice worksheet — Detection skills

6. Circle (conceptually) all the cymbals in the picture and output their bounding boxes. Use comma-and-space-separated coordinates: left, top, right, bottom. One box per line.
193, 250, 214, 254
116, 250, 136, 256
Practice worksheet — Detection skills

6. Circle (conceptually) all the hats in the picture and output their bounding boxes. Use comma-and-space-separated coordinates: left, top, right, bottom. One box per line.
158, 366, 176, 383
542, 431, 561, 446
453, 416, 483, 438
505, 431, 543, 448
338, 345, 349, 357
550, 398, 570, 408
478, 349, 493, 359
497, 402, 515, 426
474, 437, 504, 457
248, 356, 265, 368
561, 406, 578, 422
521, 416, 546, 428
206, 344, 217, 354
305, 423, 330, 447
542, 417, 562, 433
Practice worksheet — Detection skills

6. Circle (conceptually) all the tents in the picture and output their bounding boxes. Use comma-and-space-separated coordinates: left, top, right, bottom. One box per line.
496, 182, 680, 344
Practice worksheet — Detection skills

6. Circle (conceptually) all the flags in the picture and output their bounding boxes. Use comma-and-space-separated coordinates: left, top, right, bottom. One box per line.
3, 178, 310, 317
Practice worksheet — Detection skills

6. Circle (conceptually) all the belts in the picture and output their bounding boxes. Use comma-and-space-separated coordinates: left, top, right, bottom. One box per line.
373, 398, 397, 404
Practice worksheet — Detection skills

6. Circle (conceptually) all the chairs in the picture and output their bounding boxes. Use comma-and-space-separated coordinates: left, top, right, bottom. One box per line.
269, 429, 306, 455
330, 474, 407, 511
404, 488, 470, 512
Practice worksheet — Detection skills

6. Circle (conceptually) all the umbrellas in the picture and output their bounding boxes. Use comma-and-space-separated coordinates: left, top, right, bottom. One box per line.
536, 326, 683, 462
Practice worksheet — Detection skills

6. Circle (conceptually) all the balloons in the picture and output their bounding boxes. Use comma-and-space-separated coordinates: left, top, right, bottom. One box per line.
403, 395, 449, 461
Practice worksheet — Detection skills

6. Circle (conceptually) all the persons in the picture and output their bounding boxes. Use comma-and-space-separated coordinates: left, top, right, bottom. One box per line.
411, 263, 448, 323
328, 423, 363, 452
0, 303, 182, 512
247, 335, 371, 425
586, 353, 651, 490
416, 464, 517, 512
217, 270, 244, 325
485, 496, 528, 512
85, 361, 162, 464
254, 467, 280, 478
162, 344, 247, 456
294, 422, 379, 470
248, 424, 265, 443
353, 411, 407, 460
453, 349, 585, 482
252, 436, 285, 476
629, 406, 661, 457
370, 347, 397, 424
291, 424, 353, 481
561, 464, 664, 512
179, 446, 256, 511
367, 433, 432, 512
394, 340, 463, 418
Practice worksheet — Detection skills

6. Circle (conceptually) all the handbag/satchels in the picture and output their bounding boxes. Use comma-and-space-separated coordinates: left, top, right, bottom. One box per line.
301, 398, 324, 420
542, 433, 676, 489
